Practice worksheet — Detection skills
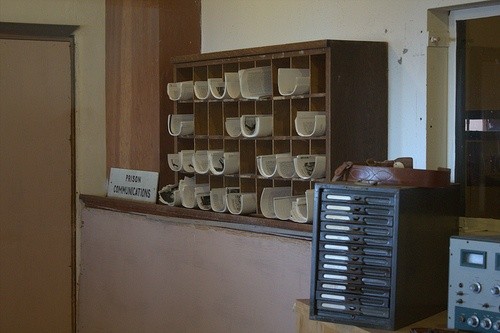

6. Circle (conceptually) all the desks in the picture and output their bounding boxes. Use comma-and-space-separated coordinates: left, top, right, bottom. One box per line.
295, 299, 476, 332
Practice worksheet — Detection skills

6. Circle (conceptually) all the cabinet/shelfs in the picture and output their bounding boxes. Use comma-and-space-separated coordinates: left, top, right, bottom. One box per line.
170, 38, 388, 222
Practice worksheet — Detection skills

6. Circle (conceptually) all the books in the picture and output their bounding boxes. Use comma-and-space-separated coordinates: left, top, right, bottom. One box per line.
148, 65, 331, 226
